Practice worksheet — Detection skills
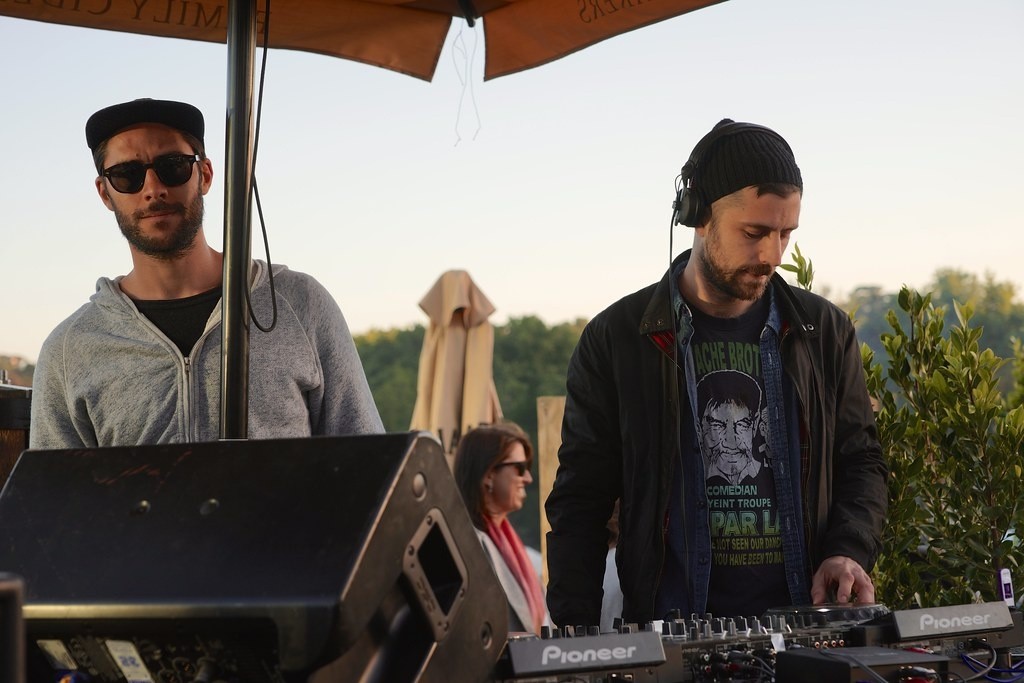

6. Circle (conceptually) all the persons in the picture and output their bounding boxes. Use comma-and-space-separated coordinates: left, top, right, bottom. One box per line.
544, 117, 893, 638
30, 98, 392, 449
446, 417, 544, 644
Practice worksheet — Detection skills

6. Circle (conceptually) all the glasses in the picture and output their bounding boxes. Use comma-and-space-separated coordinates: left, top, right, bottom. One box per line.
101, 152, 200, 194
495, 460, 530, 476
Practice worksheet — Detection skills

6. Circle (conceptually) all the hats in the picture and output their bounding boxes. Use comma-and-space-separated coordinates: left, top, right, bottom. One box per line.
85, 98, 205, 156
688, 119, 803, 201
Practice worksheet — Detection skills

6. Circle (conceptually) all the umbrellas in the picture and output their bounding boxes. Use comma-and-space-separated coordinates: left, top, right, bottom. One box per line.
0, 0, 726, 440
408, 267, 506, 478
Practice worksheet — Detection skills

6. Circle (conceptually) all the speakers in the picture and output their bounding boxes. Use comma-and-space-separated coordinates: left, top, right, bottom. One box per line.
0, 429, 509, 683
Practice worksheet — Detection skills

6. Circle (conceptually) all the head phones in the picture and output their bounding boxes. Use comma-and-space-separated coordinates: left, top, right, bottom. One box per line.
672, 122, 794, 227
